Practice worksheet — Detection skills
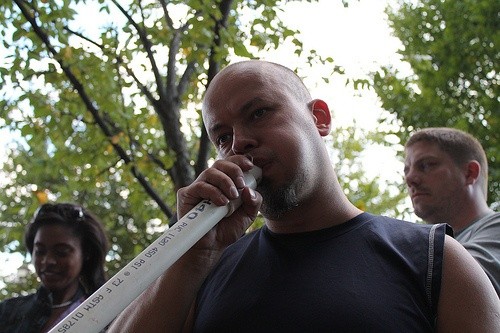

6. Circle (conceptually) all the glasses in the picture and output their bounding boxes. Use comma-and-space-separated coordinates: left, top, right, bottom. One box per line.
33, 203, 83, 222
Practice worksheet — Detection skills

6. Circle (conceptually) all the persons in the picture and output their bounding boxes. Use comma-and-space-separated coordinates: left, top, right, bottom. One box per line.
105, 59, 500, 333
1, 202, 110, 333
405, 127, 500, 301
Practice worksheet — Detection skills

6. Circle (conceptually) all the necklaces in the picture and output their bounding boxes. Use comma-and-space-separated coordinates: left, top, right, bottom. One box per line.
35, 284, 84, 309
